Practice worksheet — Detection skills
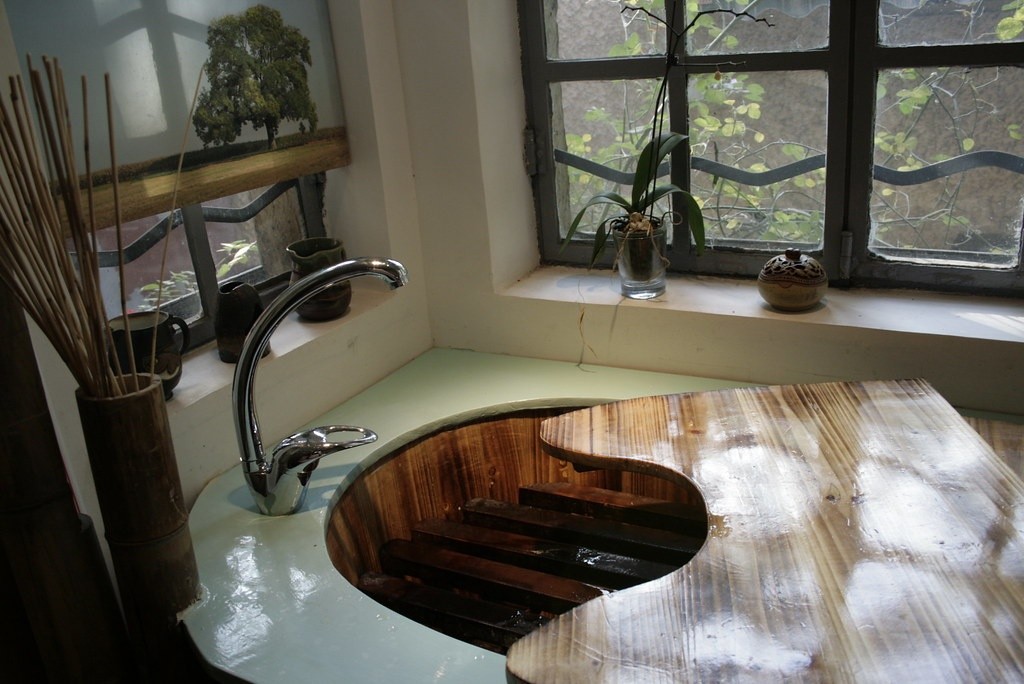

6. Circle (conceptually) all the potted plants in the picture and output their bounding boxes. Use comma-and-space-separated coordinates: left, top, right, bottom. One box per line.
557, 4, 776, 301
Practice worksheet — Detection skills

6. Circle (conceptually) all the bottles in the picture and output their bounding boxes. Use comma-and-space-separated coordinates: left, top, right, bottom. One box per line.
215, 281, 271, 366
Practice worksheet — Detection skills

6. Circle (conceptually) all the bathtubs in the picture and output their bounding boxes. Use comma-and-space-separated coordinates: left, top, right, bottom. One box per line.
179, 345, 1024, 684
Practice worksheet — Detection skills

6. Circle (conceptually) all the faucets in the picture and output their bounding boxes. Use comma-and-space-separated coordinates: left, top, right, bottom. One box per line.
230, 257, 411, 518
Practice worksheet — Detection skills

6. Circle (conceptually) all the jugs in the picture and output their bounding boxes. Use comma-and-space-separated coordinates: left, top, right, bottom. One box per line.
284, 236, 351, 320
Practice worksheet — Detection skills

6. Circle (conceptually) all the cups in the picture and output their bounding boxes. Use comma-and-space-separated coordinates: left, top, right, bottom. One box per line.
104, 309, 190, 400
